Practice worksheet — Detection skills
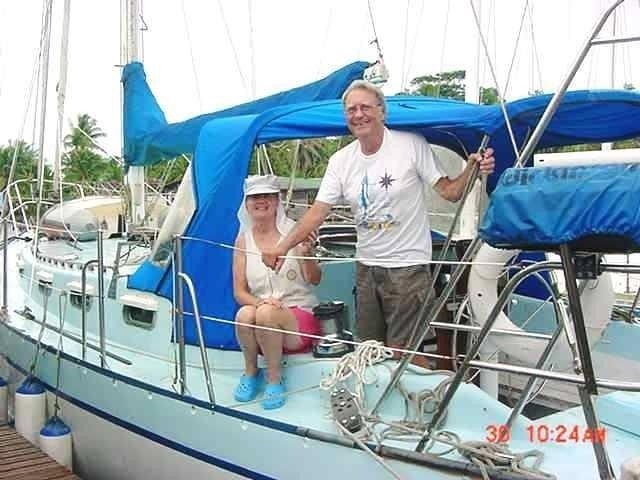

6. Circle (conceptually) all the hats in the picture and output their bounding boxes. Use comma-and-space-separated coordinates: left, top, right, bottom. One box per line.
247, 175, 280, 196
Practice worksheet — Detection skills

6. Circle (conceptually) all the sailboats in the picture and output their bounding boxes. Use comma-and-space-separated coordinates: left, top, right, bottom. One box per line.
0, 0, 639, 480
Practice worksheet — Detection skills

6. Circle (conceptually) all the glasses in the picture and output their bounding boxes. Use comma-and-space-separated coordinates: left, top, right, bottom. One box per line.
345, 104, 381, 113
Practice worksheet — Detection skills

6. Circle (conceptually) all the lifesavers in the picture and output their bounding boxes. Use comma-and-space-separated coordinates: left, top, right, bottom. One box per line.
466, 243, 614, 372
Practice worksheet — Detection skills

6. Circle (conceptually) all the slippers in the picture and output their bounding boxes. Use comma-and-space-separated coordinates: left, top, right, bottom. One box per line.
234, 369, 287, 409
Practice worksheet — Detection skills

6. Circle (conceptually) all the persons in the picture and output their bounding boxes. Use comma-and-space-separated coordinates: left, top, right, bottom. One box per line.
261, 78, 495, 370
232, 173, 322, 409
